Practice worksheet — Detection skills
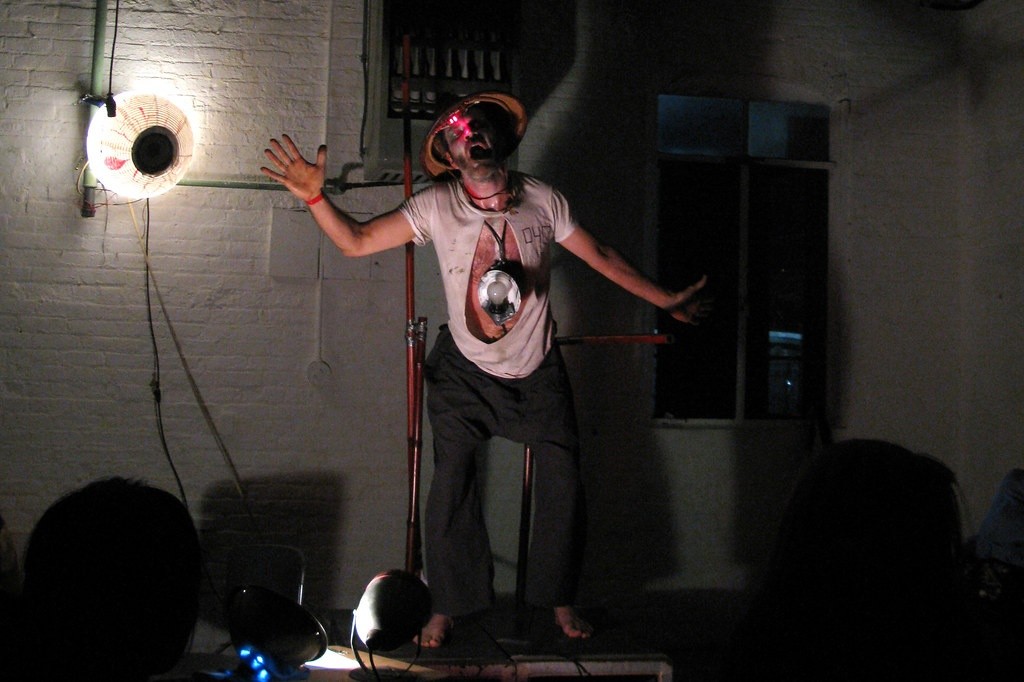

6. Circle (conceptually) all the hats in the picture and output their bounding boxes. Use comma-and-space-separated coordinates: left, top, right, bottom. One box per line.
421, 95, 528, 178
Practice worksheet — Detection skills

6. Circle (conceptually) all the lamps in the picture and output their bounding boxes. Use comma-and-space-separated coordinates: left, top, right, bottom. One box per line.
226, 592, 327, 682
477, 270, 520, 326
82, 89, 195, 199
350, 567, 434, 682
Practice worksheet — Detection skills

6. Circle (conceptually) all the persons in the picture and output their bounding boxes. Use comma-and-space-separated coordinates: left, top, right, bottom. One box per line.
722, 440, 1024, 682
0, 476, 200, 682
260, 90, 716, 648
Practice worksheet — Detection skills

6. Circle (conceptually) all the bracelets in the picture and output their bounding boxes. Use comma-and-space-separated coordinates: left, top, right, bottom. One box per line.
304, 192, 325, 205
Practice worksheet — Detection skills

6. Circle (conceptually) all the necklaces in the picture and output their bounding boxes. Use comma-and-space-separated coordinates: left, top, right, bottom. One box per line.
460, 178, 521, 327
